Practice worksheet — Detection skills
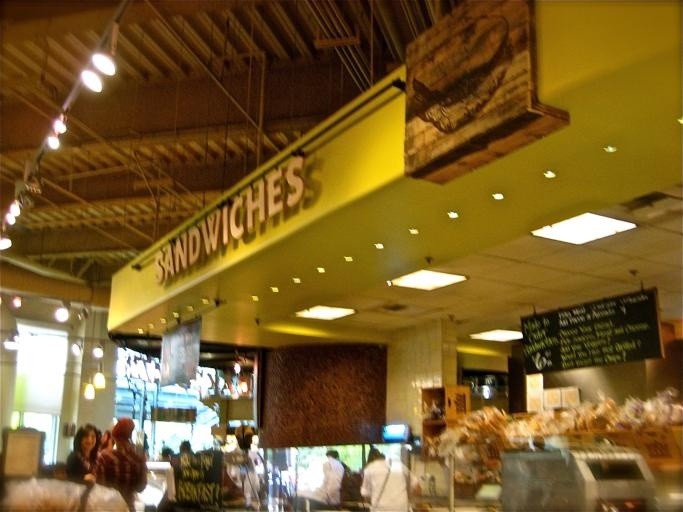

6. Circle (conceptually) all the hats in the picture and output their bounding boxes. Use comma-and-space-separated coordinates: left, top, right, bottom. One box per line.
112, 418, 135, 441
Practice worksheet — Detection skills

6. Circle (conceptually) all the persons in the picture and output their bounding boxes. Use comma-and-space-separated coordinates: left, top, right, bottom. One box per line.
313, 449, 343, 503
168, 439, 196, 468
87, 431, 101, 472
101, 427, 113, 453
359, 446, 418, 511
91, 416, 146, 512
62, 423, 97, 484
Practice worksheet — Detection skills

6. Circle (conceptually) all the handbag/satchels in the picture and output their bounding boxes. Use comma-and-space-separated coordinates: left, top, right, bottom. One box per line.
340, 463, 362, 502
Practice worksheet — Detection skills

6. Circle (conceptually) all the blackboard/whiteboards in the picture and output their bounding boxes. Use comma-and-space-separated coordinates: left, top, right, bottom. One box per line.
175, 398, 227, 512
521, 287, 661, 374
